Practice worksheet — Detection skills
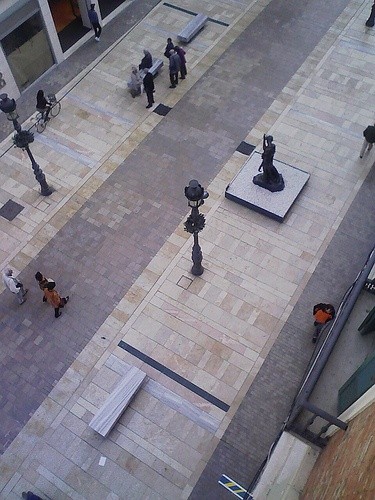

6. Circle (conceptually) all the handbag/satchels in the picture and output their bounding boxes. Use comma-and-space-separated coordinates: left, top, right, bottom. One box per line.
12, 283, 23, 294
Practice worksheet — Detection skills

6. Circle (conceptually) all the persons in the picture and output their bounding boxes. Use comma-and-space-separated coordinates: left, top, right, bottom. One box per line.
312, 303, 335, 343
35, 272, 70, 318
2, 268, 29, 305
131, 37, 187, 108
35, 90, 52, 122
258, 134, 280, 184
360, 123, 375, 159
88, 3, 102, 42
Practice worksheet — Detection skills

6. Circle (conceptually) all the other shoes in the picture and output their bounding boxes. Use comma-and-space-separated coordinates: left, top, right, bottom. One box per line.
56, 312, 62, 318
312, 338, 317, 343
23, 290, 27, 296
314, 321, 318, 326
65, 295, 69, 304
19, 299, 25, 305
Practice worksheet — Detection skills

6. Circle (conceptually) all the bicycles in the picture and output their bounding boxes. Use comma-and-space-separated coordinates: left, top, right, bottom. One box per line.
35, 99, 61, 132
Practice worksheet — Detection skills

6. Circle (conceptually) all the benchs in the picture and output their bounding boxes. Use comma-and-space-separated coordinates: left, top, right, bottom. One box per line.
89, 366, 148, 439
127, 58, 164, 88
177, 12, 208, 43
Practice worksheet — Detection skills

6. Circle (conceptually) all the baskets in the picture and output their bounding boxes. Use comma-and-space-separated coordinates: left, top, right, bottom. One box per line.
48, 94, 56, 102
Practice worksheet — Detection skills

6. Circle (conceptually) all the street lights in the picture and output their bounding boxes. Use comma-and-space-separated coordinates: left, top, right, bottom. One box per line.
183, 179, 209, 277
0, 93, 53, 196
